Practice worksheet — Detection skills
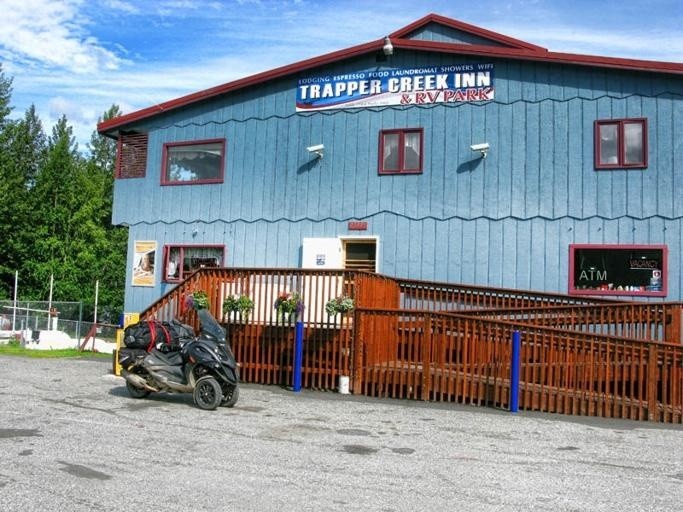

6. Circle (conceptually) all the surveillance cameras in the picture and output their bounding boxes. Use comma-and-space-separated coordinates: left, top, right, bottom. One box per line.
470, 143, 490, 153
306, 145, 325, 153
383, 45, 395, 57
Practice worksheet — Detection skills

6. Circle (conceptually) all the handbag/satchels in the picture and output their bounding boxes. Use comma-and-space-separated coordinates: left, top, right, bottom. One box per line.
124, 319, 194, 352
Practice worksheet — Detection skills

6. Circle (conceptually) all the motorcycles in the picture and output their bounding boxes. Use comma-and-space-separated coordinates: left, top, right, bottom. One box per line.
118, 295, 239, 410
